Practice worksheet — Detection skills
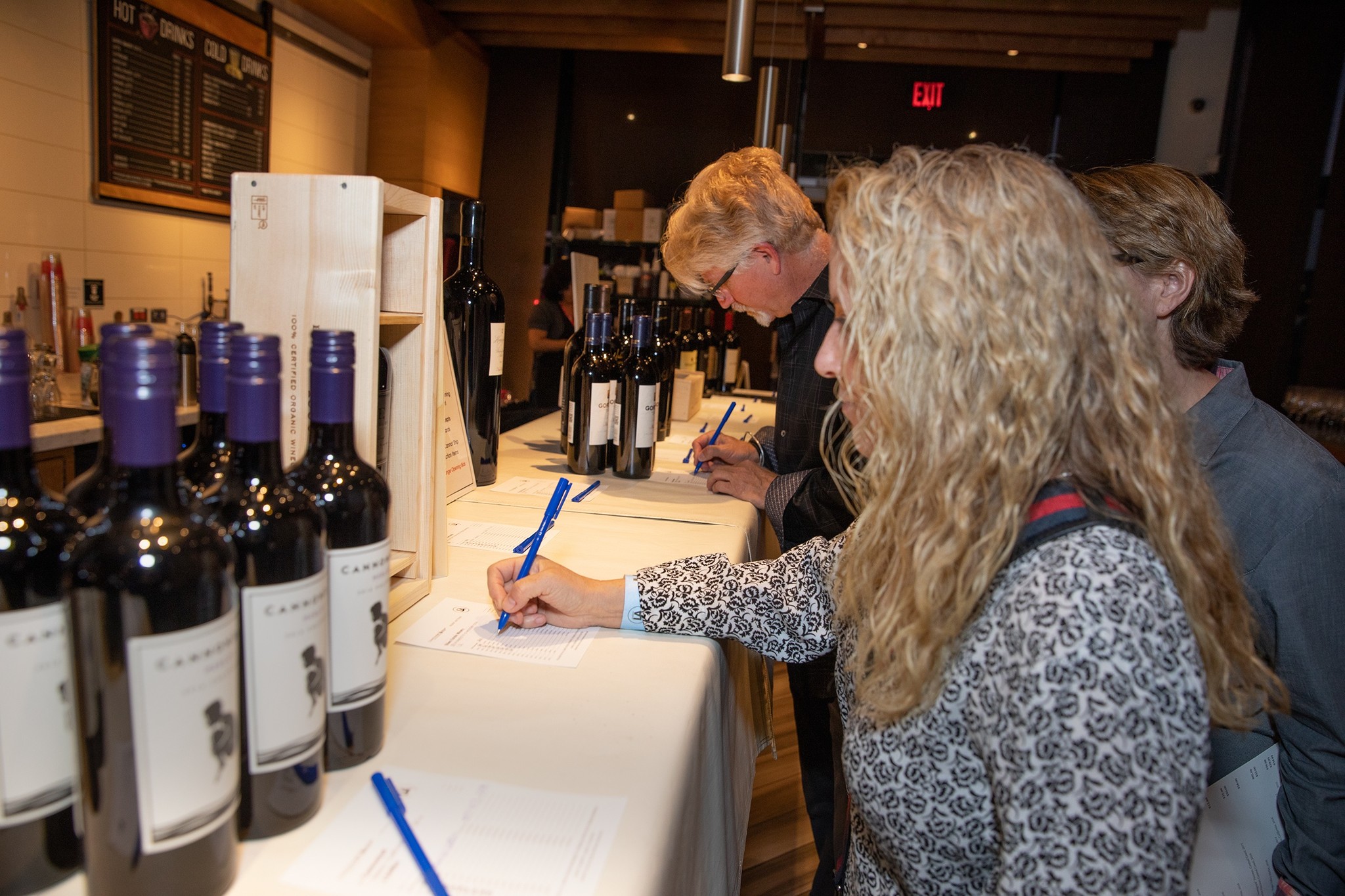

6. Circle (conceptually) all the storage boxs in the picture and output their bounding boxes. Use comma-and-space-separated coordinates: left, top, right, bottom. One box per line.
641, 208, 668, 243
600, 209, 616, 241
614, 188, 655, 210
615, 210, 643, 243
563, 205, 602, 228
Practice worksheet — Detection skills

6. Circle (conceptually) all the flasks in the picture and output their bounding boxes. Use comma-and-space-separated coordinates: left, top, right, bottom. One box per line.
178, 330, 197, 409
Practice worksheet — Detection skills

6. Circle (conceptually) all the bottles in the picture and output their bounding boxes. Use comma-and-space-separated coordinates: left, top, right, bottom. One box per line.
443, 199, 506, 487
36, 249, 95, 376
558, 283, 743, 479
0, 313, 391, 896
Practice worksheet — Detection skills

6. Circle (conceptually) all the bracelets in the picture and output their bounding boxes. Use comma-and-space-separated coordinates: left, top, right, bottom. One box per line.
743, 431, 764, 467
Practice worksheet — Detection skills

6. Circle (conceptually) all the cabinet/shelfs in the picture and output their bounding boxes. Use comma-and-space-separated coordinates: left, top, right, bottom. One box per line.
228, 169, 447, 630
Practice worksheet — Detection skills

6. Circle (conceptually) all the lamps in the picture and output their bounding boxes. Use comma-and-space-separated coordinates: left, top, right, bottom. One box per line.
775, 0, 795, 173
721, 0, 756, 86
752, 1, 780, 149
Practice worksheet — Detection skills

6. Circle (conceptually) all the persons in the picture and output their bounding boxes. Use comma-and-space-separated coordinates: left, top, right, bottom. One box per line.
660, 146, 870, 896
527, 260, 575, 422
488, 142, 1292, 896
1069, 162, 1345, 896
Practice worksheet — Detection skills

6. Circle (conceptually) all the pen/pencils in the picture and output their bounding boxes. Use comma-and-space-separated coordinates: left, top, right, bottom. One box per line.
693, 401, 737, 475
743, 415, 753, 423
572, 481, 600, 502
771, 390, 776, 400
700, 422, 708, 433
741, 404, 745, 411
753, 396, 758, 402
513, 520, 555, 553
682, 448, 693, 463
495, 477, 572, 636
370, 772, 449, 896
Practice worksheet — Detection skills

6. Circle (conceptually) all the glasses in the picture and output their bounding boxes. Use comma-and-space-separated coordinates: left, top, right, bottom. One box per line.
707, 261, 740, 300
